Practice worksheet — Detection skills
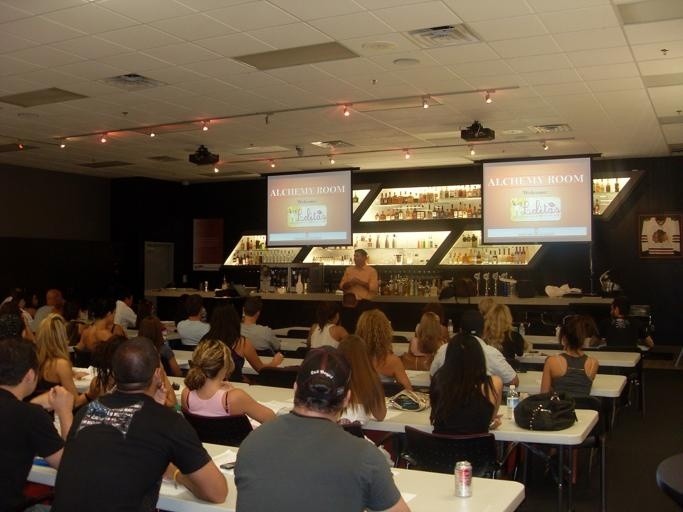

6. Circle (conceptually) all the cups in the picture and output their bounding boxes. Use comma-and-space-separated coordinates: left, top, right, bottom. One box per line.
313, 255, 353, 265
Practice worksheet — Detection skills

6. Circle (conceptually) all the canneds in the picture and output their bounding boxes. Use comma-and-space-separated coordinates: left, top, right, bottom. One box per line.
455, 461, 472, 498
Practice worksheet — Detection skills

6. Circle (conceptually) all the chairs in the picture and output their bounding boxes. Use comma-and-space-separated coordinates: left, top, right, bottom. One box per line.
549, 393, 608, 511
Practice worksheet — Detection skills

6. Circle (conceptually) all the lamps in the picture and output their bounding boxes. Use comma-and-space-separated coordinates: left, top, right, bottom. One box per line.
53, 85, 522, 149
212, 137, 577, 175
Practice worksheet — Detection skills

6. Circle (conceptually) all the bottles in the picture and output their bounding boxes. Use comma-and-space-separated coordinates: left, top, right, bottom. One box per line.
518, 323, 527, 336
593, 176, 619, 214
231, 235, 264, 265
505, 384, 519, 419
295, 272, 303, 296
363, 233, 433, 249
267, 251, 296, 263
394, 253, 429, 264
446, 245, 530, 264
379, 275, 438, 296
447, 319, 454, 336
374, 185, 481, 220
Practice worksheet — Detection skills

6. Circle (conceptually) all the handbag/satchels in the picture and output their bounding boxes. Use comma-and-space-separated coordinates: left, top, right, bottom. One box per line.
387, 389, 429, 413
515, 390, 577, 431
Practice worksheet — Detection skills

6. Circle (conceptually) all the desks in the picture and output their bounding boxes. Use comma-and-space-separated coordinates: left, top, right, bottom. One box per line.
68, 322, 644, 468
67, 367, 597, 511
28, 415, 527, 512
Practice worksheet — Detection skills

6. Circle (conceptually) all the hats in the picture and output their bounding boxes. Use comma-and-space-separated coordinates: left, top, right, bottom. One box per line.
296, 346, 353, 398
457, 310, 484, 334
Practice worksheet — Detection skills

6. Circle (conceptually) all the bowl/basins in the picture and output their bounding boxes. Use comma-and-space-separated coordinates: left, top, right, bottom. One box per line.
274, 286, 287, 295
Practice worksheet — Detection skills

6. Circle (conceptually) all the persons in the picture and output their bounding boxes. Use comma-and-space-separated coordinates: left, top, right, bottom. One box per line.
0, 285, 416, 483
232, 344, 415, 512
0, 333, 73, 512
402, 289, 654, 482
52, 332, 227, 511
337, 249, 379, 301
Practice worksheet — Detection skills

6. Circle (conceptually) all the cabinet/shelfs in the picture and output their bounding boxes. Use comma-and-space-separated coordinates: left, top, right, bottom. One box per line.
218, 169, 649, 273
